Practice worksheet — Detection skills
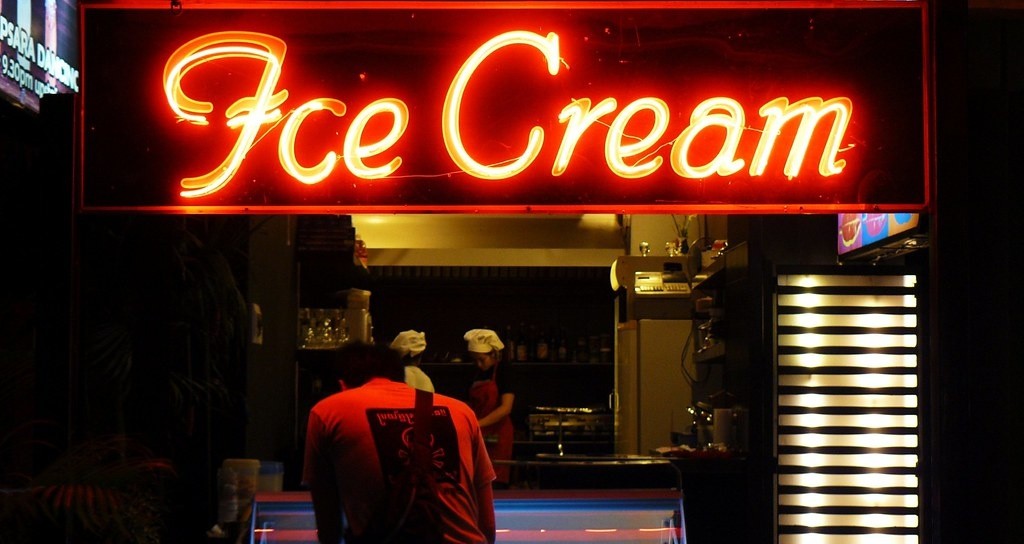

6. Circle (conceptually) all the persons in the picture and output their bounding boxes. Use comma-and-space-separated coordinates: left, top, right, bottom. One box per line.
304, 340, 496, 544
459, 329, 517, 490
391, 330, 435, 392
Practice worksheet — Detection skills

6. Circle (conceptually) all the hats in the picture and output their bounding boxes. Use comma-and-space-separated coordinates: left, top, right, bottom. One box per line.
464, 328, 504, 353
390, 330, 426, 357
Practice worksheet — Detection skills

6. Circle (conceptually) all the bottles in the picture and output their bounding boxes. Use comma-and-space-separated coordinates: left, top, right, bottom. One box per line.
482, 321, 610, 361
217, 460, 239, 524
299, 308, 348, 347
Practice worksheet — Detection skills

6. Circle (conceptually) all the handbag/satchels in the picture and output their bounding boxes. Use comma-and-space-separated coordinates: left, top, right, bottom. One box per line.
344, 484, 443, 544
